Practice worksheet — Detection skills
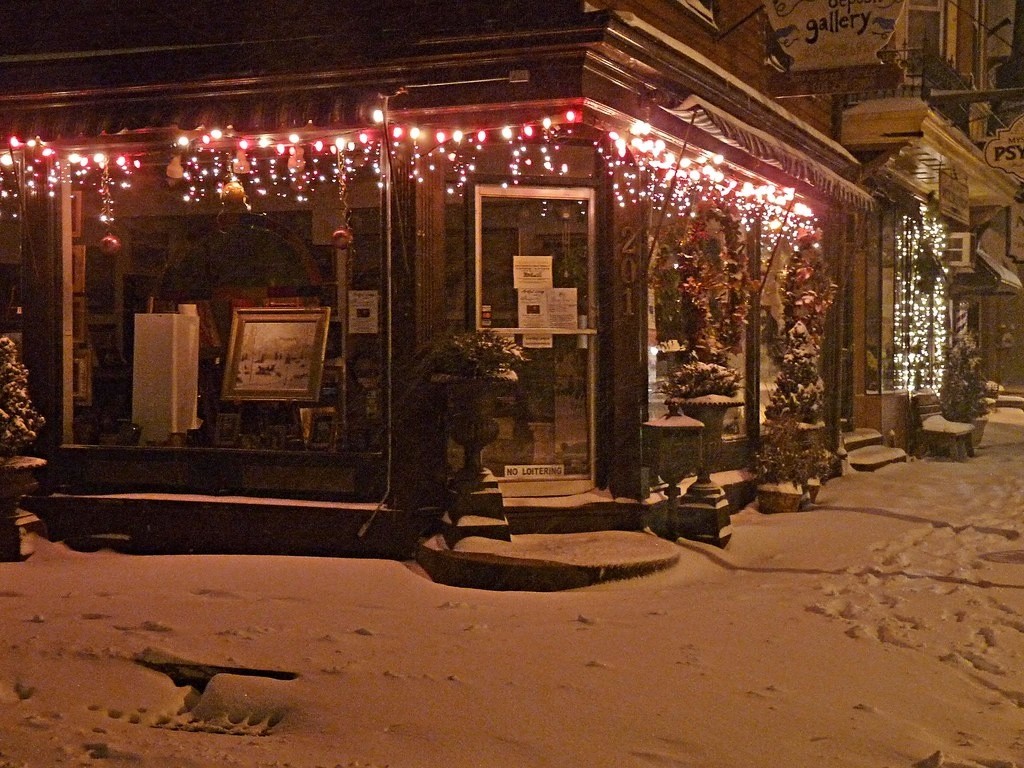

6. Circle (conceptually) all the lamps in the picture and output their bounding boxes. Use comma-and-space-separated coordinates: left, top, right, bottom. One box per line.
166, 157, 183, 178
287, 147, 306, 174
232, 151, 250, 173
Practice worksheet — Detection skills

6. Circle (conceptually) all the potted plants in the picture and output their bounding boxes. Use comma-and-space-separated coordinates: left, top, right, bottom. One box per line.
651, 361, 745, 536
765, 322, 831, 504
416, 328, 531, 549
924, 346, 989, 455
0, 337, 47, 562
747, 417, 805, 514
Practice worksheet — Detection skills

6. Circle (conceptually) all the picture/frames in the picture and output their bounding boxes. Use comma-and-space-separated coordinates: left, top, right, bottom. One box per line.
220, 306, 331, 402
306, 412, 336, 449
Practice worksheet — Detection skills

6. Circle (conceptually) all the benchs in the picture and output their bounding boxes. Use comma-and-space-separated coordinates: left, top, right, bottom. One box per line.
911, 394, 975, 462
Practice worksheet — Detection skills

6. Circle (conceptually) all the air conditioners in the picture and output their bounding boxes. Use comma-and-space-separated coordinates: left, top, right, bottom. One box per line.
947, 232, 977, 268
